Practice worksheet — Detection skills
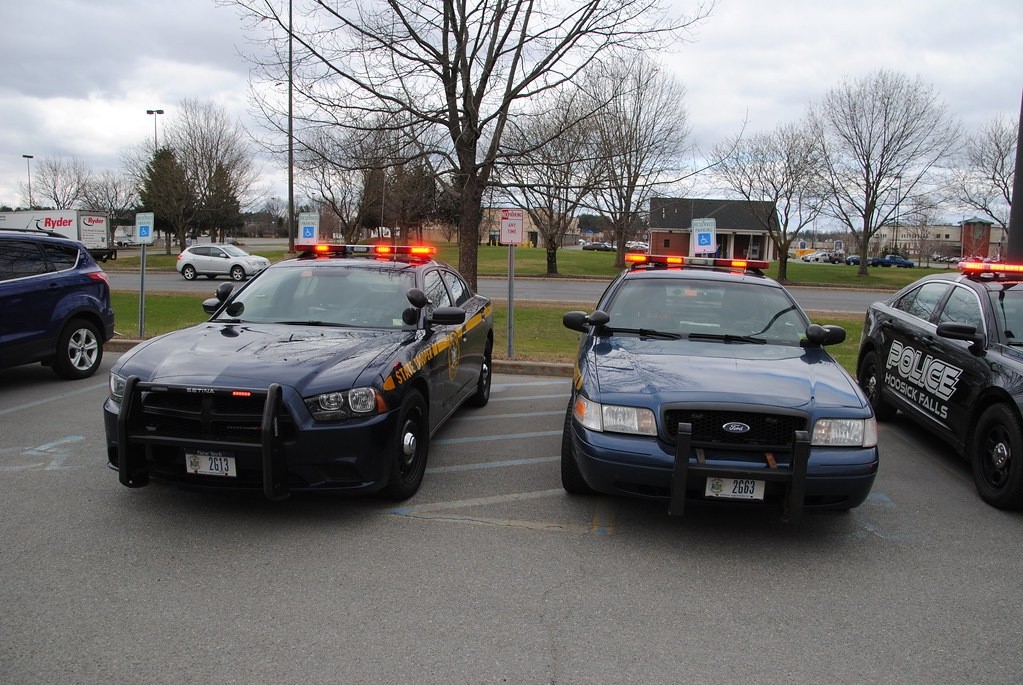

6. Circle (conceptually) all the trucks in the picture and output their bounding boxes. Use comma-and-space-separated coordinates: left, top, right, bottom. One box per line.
108, 226, 155, 248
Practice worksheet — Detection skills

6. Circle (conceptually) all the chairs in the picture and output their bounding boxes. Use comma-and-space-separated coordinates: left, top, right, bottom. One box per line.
313, 276, 356, 305
622, 284, 680, 328
720, 290, 773, 333
388, 281, 418, 322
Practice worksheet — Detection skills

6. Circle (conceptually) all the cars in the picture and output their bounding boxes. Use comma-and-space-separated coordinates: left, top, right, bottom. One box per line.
558, 253, 879, 525
176, 243, 272, 281
931, 254, 1003, 264
801, 250, 915, 269
856, 260, 1023, 512
102, 243, 494, 502
578, 238, 649, 254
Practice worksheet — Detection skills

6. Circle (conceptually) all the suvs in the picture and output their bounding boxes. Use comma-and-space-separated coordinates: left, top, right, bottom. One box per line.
0, 227, 116, 381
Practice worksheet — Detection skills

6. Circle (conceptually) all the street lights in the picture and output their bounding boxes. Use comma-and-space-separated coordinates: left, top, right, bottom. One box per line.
890, 175, 903, 269
22, 155, 33, 210
146, 109, 164, 158
